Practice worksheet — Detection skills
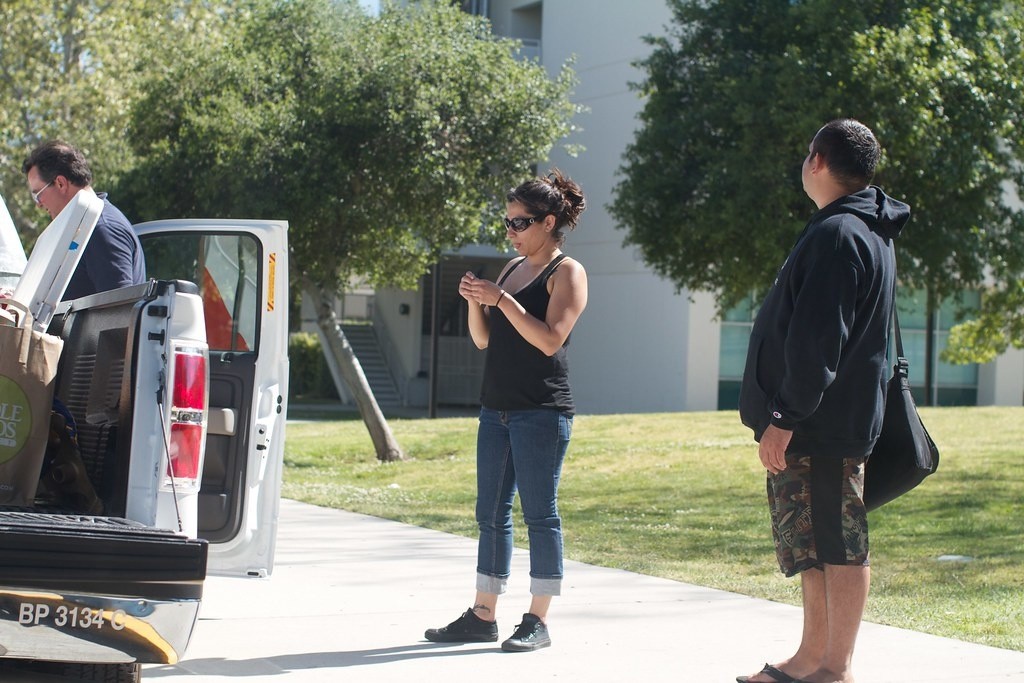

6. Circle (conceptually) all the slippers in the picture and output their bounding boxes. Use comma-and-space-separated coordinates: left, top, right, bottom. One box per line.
736, 663, 811, 683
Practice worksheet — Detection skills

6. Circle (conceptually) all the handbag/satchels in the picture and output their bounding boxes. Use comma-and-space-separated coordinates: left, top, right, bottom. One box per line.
0, 298, 65, 507
864, 373, 939, 512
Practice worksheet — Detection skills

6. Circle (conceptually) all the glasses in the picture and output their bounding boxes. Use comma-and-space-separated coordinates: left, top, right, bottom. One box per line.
503, 210, 562, 232
30, 173, 68, 204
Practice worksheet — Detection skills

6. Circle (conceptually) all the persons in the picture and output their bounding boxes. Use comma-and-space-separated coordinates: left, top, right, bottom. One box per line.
425, 167, 590, 652
5, 139, 145, 303
732, 117, 900, 683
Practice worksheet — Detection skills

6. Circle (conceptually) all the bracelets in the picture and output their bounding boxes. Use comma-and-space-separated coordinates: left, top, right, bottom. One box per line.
494, 290, 508, 307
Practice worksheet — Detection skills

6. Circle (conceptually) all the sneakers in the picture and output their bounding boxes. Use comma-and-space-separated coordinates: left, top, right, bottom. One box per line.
502, 613, 551, 651
424, 607, 499, 642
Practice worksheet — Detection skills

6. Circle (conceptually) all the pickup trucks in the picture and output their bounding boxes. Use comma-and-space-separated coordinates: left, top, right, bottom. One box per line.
0, 217, 290, 682
